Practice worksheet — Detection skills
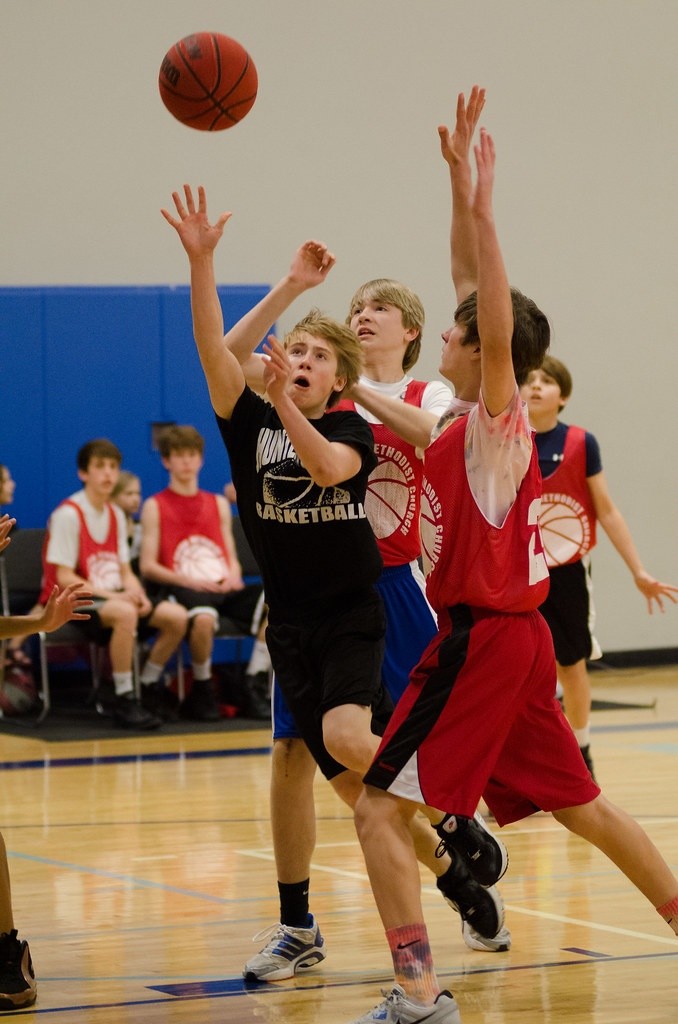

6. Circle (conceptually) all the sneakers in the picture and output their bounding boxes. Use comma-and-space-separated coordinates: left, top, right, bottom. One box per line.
445, 896, 511, 952
242, 913, 327, 981
435, 842, 504, 939
112, 696, 161, 730
140, 678, 179, 721
95, 693, 112, 715
431, 809, 509, 888
241, 670, 271, 720
352, 984, 461, 1024
188, 676, 221, 721
0, 929, 37, 1011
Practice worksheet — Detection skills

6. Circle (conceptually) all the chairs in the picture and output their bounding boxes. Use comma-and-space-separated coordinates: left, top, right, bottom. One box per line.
0, 516, 266, 726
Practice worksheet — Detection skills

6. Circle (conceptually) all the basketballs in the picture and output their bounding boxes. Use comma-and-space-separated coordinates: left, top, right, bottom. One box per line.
158, 33, 259, 131
1, 667, 36, 713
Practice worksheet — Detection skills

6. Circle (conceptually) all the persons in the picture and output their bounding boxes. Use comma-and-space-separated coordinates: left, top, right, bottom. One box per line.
355, 87, 677, 1024
224, 239, 513, 982
161, 182, 510, 939
0, 427, 271, 1009
519, 355, 678, 780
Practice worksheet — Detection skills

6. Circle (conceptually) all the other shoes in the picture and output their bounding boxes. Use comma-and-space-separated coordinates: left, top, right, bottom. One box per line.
580, 744, 593, 771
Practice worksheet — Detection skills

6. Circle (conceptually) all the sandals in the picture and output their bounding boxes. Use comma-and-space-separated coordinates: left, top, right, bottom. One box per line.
4, 647, 33, 670
